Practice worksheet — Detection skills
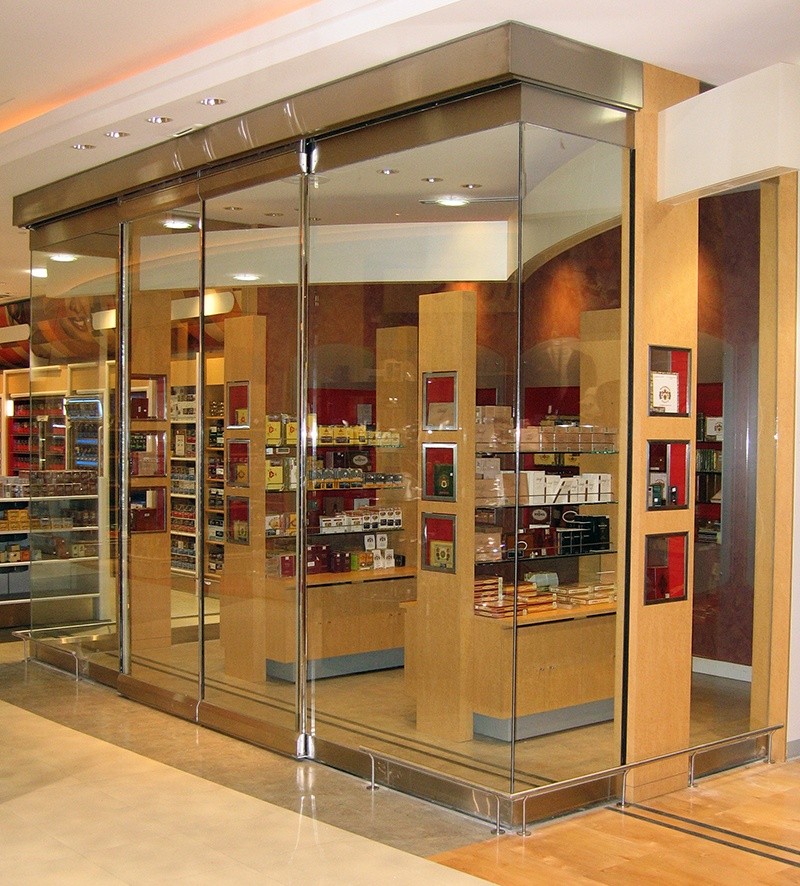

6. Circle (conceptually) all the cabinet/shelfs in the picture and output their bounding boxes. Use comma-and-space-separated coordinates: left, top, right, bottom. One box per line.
167, 423, 202, 568
204, 410, 227, 577
265, 442, 407, 575
692, 436, 724, 543
469, 440, 625, 619
71, 421, 166, 530
9, 409, 68, 475
0, 494, 100, 606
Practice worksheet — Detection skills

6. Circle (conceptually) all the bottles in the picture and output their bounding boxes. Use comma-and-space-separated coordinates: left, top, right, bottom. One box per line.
202, 458, 223, 535
130, 433, 147, 451
14, 402, 99, 470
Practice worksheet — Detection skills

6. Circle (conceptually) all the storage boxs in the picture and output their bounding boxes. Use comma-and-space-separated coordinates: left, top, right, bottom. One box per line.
0, 417, 406, 574
421, 404, 621, 564
